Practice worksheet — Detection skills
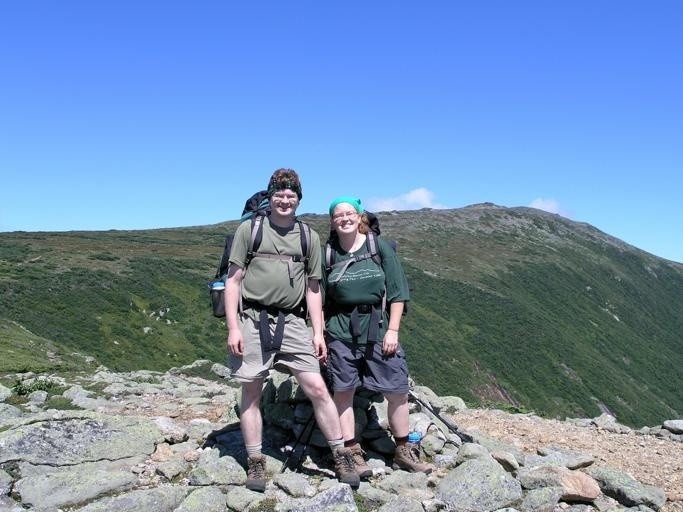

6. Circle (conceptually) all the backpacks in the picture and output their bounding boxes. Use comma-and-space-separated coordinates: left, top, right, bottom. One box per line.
325, 210, 408, 316
207, 191, 310, 318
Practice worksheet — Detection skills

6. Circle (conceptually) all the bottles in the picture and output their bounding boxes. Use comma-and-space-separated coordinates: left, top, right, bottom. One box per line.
211, 282, 225, 317
408, 431, 420, 457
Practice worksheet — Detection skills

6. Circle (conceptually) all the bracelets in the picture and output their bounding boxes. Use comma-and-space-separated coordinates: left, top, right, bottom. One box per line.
388, 328, 398, 333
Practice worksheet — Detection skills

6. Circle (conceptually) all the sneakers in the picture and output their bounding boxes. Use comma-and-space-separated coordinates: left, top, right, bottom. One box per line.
247, 454, 266, 491
392, 441, 432, 475
331, 443, 372, 486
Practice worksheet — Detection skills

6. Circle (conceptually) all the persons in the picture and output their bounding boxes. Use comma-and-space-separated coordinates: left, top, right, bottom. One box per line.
318, 196, 433, 477
223, 167, 359, 492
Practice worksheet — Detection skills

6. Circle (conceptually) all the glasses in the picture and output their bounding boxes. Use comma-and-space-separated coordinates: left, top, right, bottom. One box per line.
333, 211, 358, 221
